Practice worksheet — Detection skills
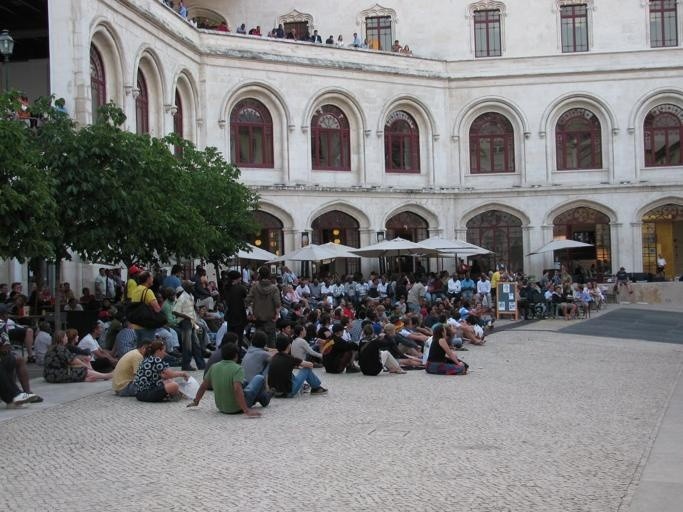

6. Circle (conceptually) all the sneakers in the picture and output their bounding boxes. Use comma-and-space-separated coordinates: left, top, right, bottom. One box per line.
302, 383, 328, 395
7, 392, 44, 409
346, 364, 360, 373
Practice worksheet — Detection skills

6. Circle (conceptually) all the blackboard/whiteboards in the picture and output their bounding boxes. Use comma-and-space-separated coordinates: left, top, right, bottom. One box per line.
497, 282, 517, 314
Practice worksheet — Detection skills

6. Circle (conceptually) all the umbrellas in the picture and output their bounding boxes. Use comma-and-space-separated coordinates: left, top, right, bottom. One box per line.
224, 235, 494, 277
525, 239, 595, 271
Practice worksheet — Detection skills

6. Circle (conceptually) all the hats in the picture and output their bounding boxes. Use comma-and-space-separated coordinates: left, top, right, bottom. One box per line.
129, 264, 141, 274
182, 280, 197, 289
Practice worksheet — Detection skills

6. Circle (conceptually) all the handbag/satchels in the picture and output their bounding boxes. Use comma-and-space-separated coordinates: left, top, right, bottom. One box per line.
127, 288, 168, 329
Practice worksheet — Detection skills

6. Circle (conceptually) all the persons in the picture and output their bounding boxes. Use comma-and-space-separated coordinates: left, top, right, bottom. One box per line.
654, 253, 667, 280
1, 259, 634, 416
56, 97, 69, 114
163, 0, 414, 55
